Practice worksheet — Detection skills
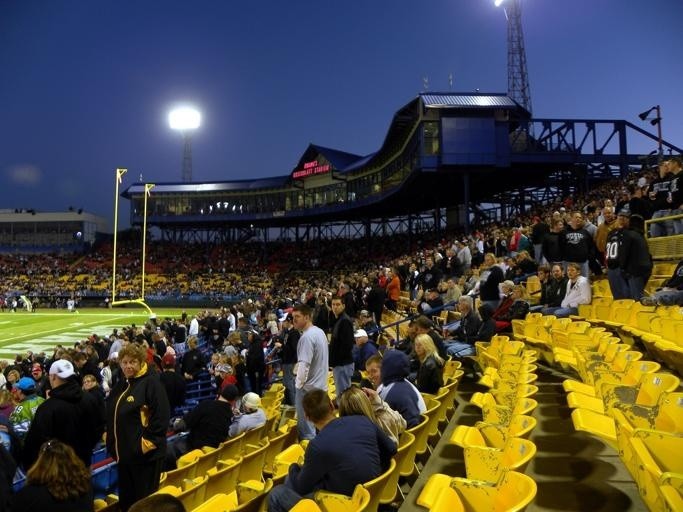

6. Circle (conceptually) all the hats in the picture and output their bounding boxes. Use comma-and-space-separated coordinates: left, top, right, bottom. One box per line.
354, 329, 369, 337
49, 359, 76, 379
242, 391, 262, 410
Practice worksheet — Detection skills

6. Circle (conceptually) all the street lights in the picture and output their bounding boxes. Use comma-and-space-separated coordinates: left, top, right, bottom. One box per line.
494, 0, 534, 138
166, 104, 204, 186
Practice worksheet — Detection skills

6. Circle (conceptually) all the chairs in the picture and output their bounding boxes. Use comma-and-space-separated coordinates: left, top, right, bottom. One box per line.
92, 334, 552, 512
3, 269, 276, 294
510, 214, 681, 511
